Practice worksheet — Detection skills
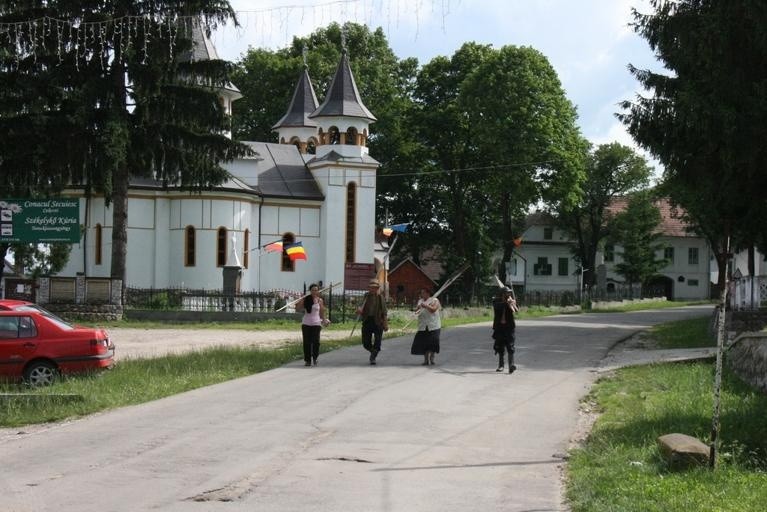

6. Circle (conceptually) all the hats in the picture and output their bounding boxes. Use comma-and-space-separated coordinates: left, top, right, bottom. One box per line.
368, 279, 381, 288
499, 286, 512, 294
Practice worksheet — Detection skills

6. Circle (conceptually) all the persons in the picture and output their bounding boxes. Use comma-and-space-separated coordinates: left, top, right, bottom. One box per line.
356, 280, 389, 364
493, 285, 519, 373
296, 285, 329, 366
411, 287, 442, 365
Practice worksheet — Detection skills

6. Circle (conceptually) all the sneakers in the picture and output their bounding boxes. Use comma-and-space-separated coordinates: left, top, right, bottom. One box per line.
371, 359, 376, 365
496, 366, 503, 371
509, 365, 516, 373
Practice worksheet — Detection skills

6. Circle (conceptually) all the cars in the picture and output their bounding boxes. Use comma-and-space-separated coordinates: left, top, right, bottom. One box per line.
1, 300, 115, 391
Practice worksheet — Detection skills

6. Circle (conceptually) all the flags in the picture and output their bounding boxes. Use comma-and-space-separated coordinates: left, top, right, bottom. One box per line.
284, 242, 307, 262
264, 241, 283, 252
513, 236, 524, 246
